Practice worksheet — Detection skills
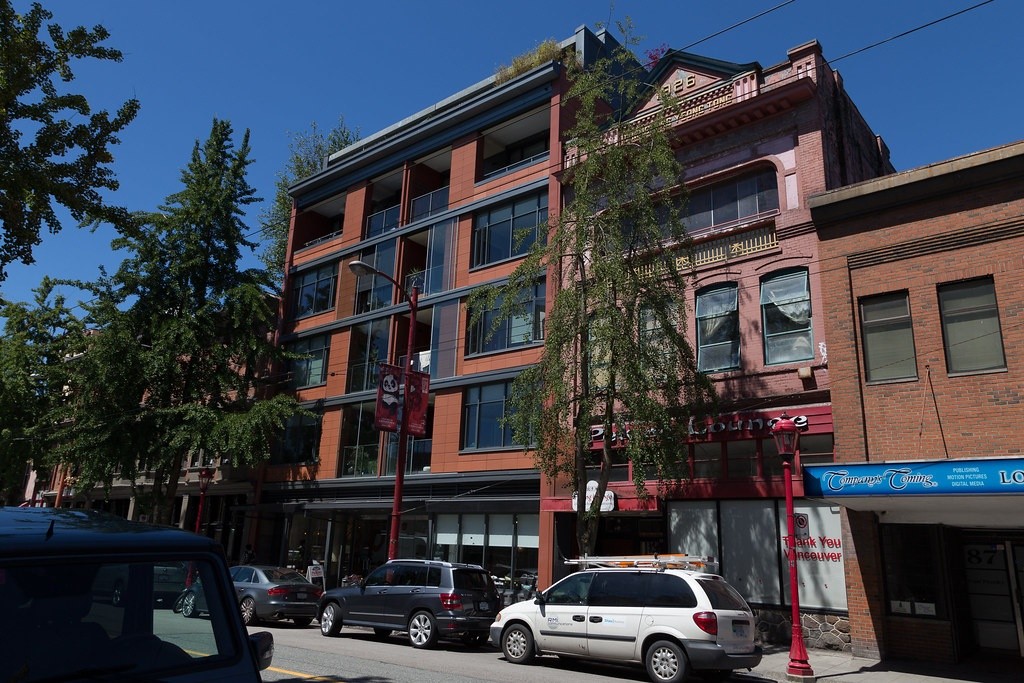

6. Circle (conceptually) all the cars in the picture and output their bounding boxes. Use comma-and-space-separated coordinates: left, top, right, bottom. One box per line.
89, 561, 188, 608
181, 565, 324, 627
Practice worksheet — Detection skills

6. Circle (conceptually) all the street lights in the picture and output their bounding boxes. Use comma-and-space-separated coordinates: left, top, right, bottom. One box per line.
769, 412, 816, 676
186, 466, 214, 587
346, 260, 423, 584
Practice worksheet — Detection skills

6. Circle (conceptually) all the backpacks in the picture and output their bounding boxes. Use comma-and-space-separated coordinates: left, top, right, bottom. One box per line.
244, 550, 254, 564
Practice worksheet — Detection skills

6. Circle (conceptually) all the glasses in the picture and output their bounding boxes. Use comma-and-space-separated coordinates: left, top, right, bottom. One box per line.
62, 592, 93, 613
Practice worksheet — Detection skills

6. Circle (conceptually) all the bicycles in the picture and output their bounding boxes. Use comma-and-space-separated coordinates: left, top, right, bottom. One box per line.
172, 571, 200, 614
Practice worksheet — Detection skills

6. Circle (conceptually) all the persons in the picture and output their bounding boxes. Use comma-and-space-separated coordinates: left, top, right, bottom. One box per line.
239, 543, 256, 566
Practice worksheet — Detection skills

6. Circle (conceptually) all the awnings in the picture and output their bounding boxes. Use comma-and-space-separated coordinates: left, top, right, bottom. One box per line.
544, 495, 666, 512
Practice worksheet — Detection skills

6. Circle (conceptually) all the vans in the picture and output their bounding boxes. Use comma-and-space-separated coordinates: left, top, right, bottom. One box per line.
0, 502, 277, 683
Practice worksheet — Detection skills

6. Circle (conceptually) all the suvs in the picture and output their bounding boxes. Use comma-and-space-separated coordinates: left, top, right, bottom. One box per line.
314, 558, 503, 651
485, 554, 763, 683
